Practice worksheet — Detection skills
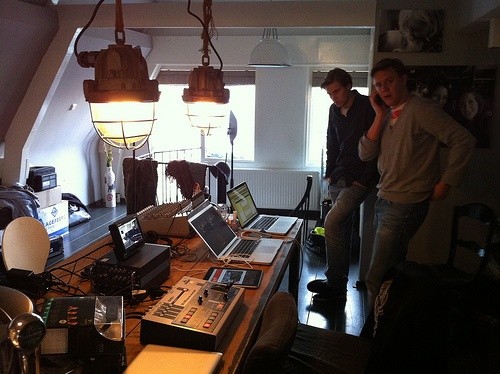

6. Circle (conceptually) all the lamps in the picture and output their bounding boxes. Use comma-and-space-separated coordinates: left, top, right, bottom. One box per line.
183, 0, 230, 137
247, 28, 291, 68
76, 0, 161, 150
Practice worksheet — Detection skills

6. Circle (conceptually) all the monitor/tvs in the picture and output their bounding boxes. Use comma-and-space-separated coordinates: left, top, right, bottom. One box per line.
108, 214, 145, 262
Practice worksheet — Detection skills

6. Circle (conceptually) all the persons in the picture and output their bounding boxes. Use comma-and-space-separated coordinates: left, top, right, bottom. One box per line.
378, 9, 443, 53
358, 58, 478, 302
306, 67, 378, 303
425, 81, 490, 146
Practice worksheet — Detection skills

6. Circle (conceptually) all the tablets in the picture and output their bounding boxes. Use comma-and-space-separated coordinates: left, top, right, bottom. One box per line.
203, 267, 264, 289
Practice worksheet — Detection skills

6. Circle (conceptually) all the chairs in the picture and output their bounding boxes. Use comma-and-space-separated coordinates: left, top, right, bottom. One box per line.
2, 217, 50, 274
449, 202, 496, 276
241, 291, 299, 374
281, 267, 422, 374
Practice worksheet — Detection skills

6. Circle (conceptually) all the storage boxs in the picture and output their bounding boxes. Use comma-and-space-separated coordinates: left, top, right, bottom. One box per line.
40, 296, 127, 374
34, 185, 70, 242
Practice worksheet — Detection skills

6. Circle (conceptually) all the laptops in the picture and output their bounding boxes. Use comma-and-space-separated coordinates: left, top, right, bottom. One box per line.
187, 204, 283, 266
226, 181, 299, 236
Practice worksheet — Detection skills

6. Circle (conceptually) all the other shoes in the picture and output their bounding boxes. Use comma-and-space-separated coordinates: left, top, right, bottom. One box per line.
312, 286, 347, 302
306, 279, 330, 293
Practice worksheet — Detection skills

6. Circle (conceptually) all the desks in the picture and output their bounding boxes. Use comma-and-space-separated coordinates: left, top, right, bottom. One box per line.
0, 214, 304, 374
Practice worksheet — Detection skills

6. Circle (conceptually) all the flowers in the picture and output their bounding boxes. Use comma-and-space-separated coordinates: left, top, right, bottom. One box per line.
103, 142, 113, 167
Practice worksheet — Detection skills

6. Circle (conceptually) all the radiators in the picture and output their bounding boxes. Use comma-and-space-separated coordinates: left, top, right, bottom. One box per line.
226, 169, 320, 210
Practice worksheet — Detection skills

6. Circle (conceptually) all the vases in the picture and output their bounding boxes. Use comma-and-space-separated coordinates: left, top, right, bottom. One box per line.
104, 167, 116, 207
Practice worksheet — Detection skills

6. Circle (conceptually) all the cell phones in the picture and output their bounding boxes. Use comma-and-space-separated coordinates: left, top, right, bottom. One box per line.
376, 94, 383, 105
